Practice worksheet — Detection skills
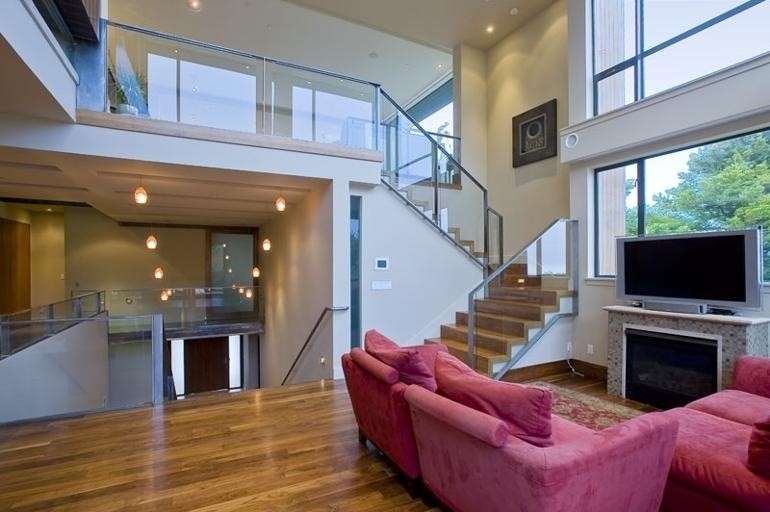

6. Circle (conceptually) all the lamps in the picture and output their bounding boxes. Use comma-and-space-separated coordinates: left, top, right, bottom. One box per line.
238, 195, 286, 298
133, 173, 169, 304
222, 241, 236, 291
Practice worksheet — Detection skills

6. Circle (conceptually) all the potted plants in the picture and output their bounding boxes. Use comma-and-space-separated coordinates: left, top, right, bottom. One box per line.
104, 60, 154, 119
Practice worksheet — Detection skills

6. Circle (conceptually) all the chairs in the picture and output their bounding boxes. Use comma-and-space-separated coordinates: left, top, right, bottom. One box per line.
403, 380, 681, 511
339, 342, 450, 500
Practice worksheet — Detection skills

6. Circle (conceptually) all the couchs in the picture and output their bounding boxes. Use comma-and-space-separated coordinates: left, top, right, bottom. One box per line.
644, 349, 770, 511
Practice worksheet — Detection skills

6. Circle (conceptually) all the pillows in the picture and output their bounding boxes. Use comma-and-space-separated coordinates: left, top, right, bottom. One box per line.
745, 410, 770, 477
361, 325, 441, 394
431, 347, 558, 451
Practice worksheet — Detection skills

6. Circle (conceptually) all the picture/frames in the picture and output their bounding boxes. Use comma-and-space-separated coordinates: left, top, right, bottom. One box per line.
511, 98, 558, 169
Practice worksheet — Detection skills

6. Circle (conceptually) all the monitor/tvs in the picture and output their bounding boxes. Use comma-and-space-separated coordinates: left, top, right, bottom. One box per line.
615, 227, 761, 314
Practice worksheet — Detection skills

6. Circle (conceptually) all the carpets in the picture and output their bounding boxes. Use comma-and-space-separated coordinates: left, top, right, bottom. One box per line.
521, 379, 646, 432
